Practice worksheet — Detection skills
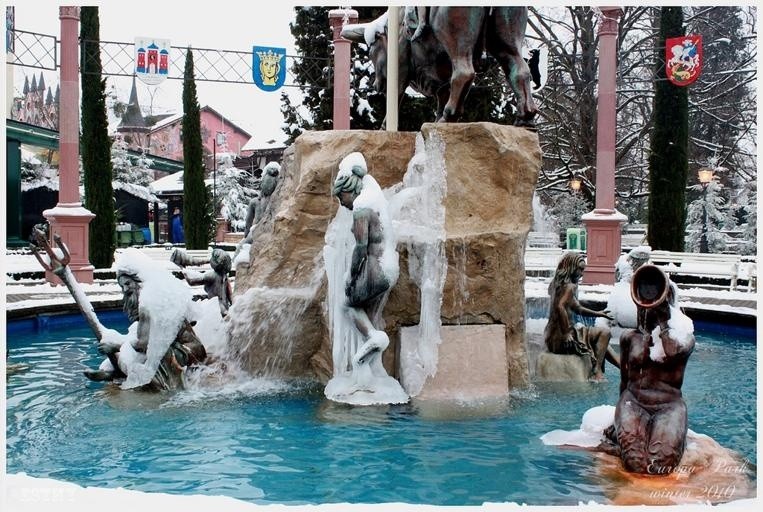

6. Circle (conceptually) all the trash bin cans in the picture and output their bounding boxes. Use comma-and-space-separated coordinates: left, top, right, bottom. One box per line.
112, 222, 151, 249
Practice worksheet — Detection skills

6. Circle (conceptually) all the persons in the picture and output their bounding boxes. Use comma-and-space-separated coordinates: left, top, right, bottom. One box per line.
409, 6, 428, 43
541, 248, 624, 383
255, 49, 284, 86
78, 273, 205, 395
147, 207, 154, 222
168, 206, 185, 246
240, 166, 278, 237
590, 281, 695, 478
330, 160, 401, 382
170, 249, 234, 320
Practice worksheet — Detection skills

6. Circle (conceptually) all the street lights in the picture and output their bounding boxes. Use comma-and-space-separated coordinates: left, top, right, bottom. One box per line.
209, 137, 226, 243
569, 174, 583, 230
698, 157, 714, 254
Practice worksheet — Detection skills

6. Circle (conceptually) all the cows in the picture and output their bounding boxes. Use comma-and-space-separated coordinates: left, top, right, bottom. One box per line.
340, 7, 536, 130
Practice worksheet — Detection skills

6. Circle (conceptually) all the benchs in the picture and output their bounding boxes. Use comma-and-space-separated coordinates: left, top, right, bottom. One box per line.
525, 230, 756, 294
